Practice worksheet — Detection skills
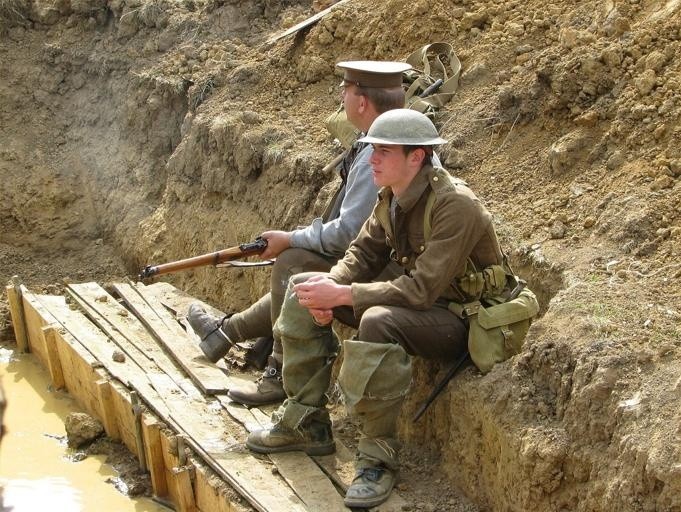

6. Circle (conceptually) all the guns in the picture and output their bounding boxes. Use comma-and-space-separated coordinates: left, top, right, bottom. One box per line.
138, 237, 267, 280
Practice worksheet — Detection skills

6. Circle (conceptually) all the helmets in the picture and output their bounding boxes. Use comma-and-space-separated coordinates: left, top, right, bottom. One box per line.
357, 109, 447, 146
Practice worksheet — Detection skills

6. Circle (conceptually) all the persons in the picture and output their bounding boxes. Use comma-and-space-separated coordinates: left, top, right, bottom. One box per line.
188, 61, 443, 405
246, 108, 503, 508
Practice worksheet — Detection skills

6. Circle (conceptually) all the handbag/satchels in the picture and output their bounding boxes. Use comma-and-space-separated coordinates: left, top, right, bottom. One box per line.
324, 41, 462, 149
423, 177, 539, 373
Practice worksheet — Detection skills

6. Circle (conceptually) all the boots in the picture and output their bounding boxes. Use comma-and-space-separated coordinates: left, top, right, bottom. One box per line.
189, 301, 235, 363
227, 355, 287, 406
246, 421, 336, 456
343, 465, 400, 507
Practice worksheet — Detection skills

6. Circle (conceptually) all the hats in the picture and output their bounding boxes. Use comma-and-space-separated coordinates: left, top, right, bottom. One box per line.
336, 61, 412, 86
